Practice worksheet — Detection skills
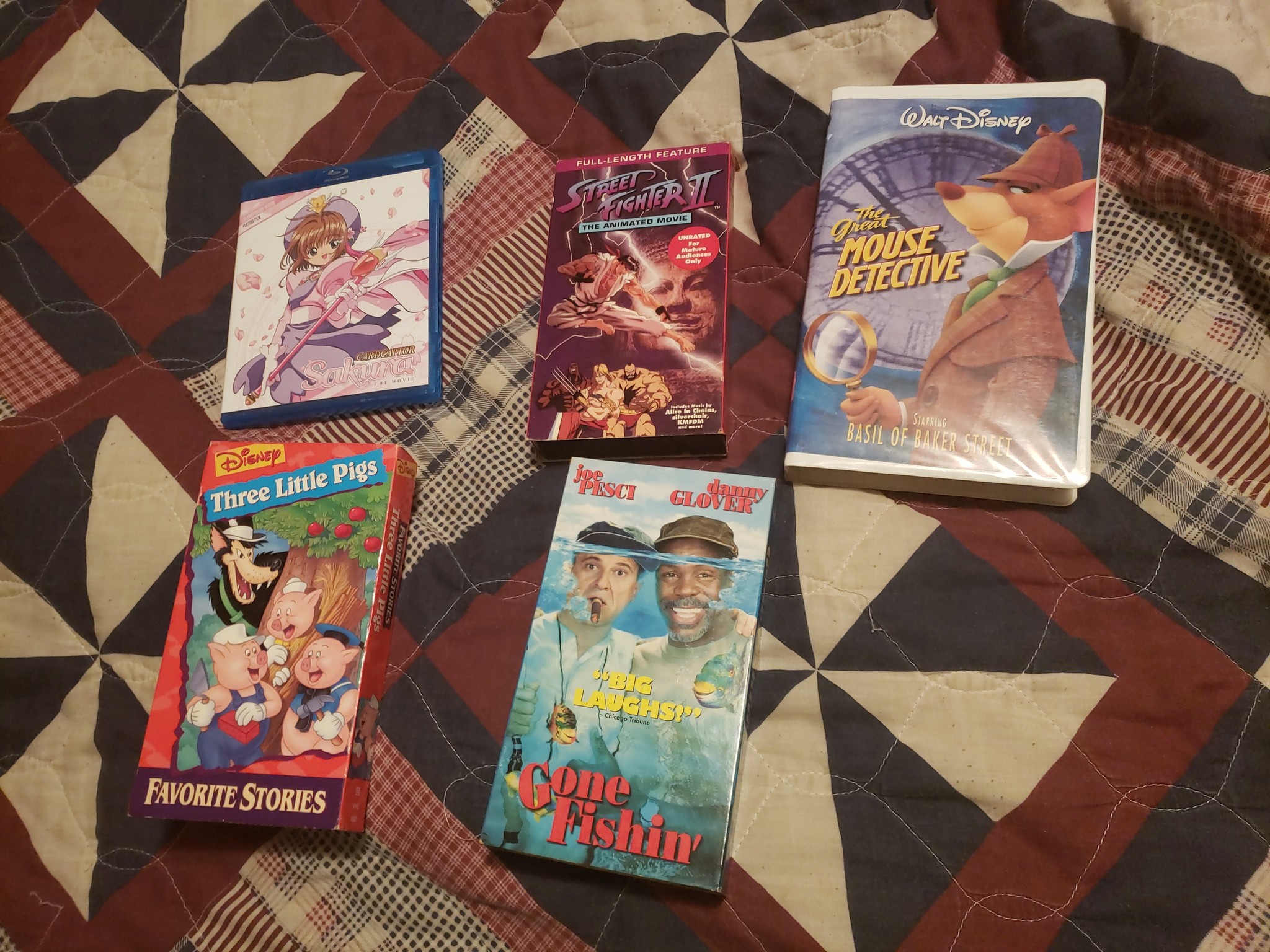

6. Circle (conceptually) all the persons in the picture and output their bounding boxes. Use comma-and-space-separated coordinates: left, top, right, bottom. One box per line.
484, 515, 756, 890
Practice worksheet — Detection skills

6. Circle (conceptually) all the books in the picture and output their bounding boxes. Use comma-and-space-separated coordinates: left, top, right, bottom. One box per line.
526, 139, 735, 462
220, 149, 444, 429
784, 79, 1106, 509
476, 453, 776, 892
126, 442, 419, 829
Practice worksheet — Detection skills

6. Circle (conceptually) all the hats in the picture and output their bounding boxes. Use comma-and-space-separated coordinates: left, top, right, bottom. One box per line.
652, 516, 739, 561
577, 518, 661, 572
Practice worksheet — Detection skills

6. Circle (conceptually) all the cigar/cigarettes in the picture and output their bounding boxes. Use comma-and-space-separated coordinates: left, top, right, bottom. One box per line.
590, 599, 602, 624
